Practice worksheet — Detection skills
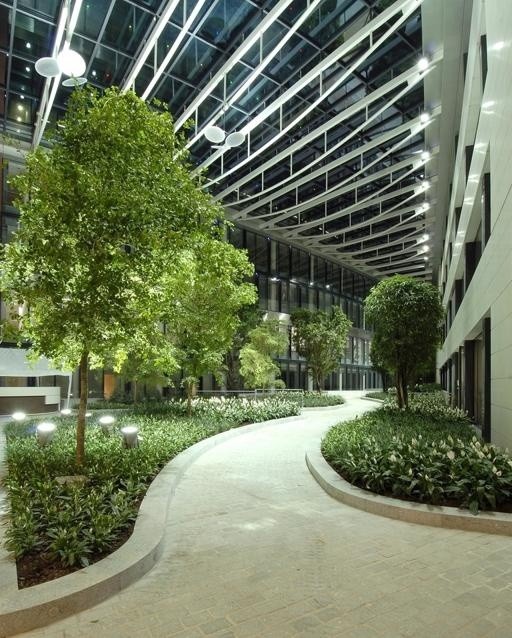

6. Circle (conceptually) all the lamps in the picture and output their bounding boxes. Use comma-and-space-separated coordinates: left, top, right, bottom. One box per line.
36, 422, 56, 446
34, 0, 87, 86
120, 425, 141, 446
204, 100, 245, 149
